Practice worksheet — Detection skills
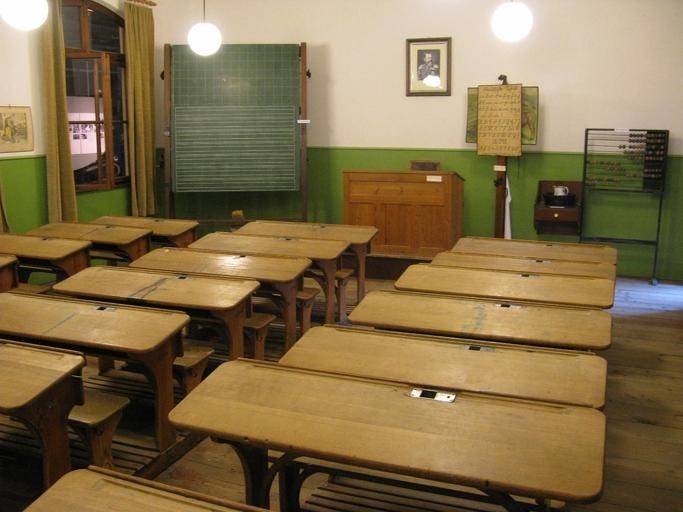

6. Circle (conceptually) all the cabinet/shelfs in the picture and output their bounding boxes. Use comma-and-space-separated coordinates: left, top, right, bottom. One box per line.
342, 170, 464, 282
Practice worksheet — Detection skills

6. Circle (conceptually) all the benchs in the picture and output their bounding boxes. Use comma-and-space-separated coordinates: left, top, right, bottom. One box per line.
66, 387, 131, 469
88, 249, 126, 261
191, 310, 277, 362
305, 267, 355, 324
86, 340, 215, 397
86, 465, 271, 512
11, 283, 51, 289
267, 447, 565, 512
252, 287, 321, 336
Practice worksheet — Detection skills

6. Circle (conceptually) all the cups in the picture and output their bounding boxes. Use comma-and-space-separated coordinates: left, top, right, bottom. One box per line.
553, 185, 568, 197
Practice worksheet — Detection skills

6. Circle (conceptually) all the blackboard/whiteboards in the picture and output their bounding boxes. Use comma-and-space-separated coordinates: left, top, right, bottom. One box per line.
169, 44, 301, 193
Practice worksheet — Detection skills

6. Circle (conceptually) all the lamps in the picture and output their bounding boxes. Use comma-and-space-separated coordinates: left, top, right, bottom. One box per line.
489, 0, 534, 43
187, 1, 223, 58
1, 0, 49, 31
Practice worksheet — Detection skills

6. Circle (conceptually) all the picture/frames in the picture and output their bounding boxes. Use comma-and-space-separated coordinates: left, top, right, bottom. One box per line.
405, 37, 452, 97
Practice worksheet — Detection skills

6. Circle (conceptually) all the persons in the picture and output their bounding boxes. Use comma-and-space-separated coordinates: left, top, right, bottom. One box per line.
417, 52, 440, 81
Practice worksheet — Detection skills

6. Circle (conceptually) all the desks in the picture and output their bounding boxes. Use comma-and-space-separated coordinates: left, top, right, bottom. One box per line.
188, 230, 351, 324
167, 359, 607, 512
0, 338, 89, 493
0, 293, 193, 454
0, 232, 94, 283
233, 220, 380, 305
279, 327, 606, 410
25, 222, 153, 261
0, 252, 21, 296
89, 216, 199, 265
128, 246, 314, 354
430, 251, 616, 280
349, 289, 613, 350
52, 266, 261, 376
394, 264, 615, 310
22, 468, 240, 512
451, 236, 616, 264
